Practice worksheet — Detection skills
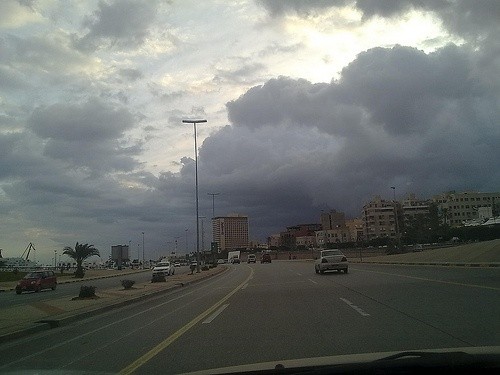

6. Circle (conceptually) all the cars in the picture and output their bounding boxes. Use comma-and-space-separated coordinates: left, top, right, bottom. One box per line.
314, 249, 348, 275
16, 270, 57, 295
151, 262, 176, 276
174, 262, 181, 267
217, 259, 224, 264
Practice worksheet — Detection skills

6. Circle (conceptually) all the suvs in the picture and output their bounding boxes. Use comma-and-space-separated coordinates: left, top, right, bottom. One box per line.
261, 254, 271, 264
190, 261, 197, 268
247, 254, 257, 264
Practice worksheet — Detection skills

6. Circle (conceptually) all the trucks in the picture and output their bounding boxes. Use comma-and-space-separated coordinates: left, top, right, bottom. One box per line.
227, 251, 240, 264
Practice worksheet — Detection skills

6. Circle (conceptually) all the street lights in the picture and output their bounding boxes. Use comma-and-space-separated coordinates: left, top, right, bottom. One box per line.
390, 187, 401, 246
54, 249, 57, 271
182, 119, 208, 272
209, 193, 218, 217
141, 232, 145, 270
197, 216, 206, 251
